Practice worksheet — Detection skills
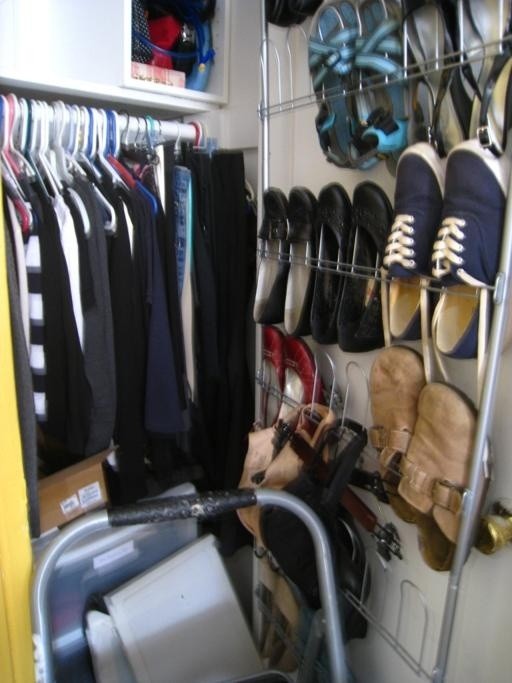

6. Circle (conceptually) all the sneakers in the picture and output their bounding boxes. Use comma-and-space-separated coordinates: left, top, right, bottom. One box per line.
379, 139, 506, 361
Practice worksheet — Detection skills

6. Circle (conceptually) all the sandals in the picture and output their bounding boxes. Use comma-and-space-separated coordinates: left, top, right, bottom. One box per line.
307, 1, 512, 169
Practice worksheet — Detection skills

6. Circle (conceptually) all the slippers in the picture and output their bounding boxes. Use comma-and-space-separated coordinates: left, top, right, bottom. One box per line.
366, 344, 493, 572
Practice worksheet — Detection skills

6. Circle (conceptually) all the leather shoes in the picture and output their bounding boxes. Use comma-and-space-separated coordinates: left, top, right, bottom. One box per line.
250, 182, 391, 353
258, 325, 323, 433
269, 575, 304, 670
254, 551, 277, 655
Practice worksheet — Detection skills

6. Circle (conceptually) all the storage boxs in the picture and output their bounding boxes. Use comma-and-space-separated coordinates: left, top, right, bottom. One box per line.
36, 439, 200, 660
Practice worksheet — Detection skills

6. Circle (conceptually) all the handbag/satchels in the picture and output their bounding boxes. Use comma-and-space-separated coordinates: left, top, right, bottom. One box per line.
257, 474, 371, 640
235, 424, 312, 539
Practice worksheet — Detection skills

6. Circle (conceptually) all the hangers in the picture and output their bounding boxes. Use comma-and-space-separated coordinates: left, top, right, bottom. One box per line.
0, 89, 254, 239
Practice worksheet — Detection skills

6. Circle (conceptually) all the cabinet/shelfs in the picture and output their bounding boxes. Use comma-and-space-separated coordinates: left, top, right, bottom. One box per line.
0, 0, 233, 114
